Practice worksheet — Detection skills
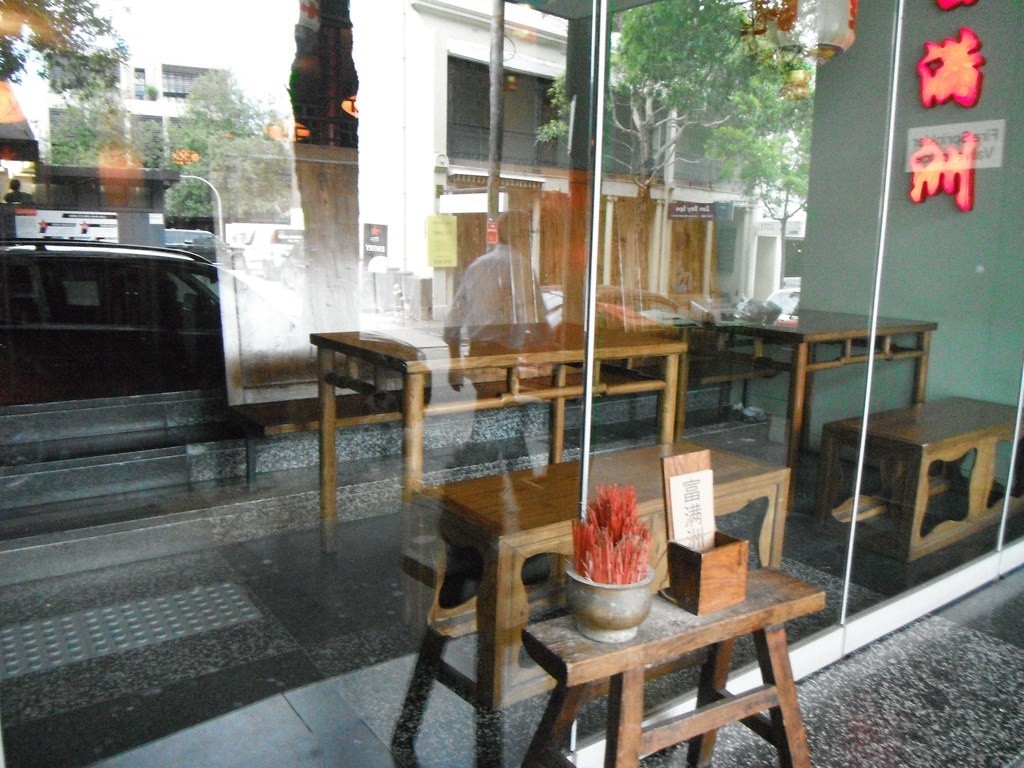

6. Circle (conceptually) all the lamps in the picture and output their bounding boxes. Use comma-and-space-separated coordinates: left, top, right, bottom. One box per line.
433, 154, 450, 196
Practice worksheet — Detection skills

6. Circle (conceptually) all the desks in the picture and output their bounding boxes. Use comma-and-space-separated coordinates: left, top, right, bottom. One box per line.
311, 322, 690, 592
671, 306, 939, 517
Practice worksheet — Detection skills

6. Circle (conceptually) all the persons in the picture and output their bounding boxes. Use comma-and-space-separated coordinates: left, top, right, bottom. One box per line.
445, 208, 548, 476
4, 179, 32, 206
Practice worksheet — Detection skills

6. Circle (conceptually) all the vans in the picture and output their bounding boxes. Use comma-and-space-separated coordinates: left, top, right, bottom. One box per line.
0, 235, 437, 503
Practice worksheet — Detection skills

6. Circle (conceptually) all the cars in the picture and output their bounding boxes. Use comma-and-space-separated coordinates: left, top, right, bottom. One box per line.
538, 275, 804, 332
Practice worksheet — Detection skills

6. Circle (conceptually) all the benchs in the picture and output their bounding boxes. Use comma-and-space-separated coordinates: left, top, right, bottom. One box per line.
227, 347, 1024, 768
